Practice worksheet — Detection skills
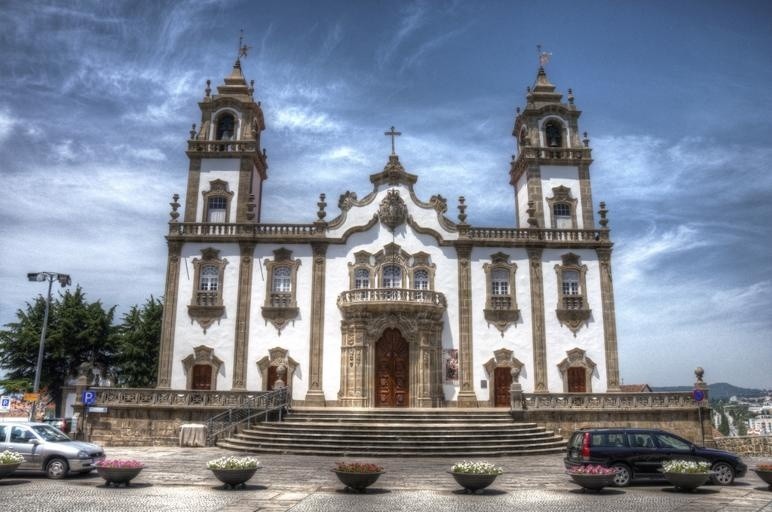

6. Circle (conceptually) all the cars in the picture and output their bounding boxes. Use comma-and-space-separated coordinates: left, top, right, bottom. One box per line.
43, 416, 71, 434
0, 417, 108, 482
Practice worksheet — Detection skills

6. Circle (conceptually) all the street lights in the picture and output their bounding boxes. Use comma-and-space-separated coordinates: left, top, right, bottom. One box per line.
22, 270, 74, 420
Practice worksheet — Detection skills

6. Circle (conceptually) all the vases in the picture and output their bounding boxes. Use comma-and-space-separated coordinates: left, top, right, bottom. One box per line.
0, 463, 21, 479
445, 470, 497, 495
205, 467, 264, 490
656, 468, 717, 491
565, 472, 617, 492
748, 468, 772, 491
90, 463, 149, 488
330, 469, 387, 492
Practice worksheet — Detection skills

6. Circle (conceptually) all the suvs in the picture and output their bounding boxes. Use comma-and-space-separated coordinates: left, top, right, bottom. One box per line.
564, 424, 750, 489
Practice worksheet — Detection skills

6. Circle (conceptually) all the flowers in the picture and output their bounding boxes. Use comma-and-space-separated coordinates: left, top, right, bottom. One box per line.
0, 450, 25, 465
333, 460, 384, 473
205, 455, 261, 470
755, 463, 772, 471
660, 458, 712, 473
94, 459, 144, 469
568, 462, 617, 475
450, 459, 504, 474
